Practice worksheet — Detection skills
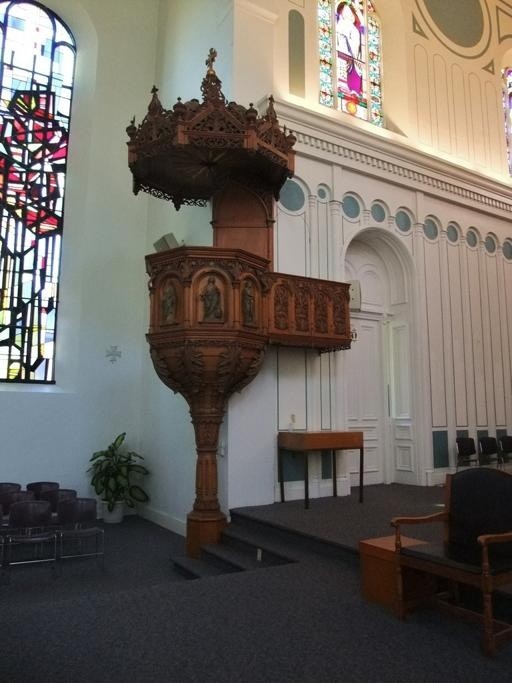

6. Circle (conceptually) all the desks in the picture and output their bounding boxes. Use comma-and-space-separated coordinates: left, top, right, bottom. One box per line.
277, 431, 364, 509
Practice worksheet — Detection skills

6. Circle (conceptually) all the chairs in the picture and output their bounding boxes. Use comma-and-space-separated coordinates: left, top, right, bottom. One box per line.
480, 437, 502, 470
0, 481, 104, 568
390, 466, 512, 658
455, 438, 477, 474
500, 436, 512, 470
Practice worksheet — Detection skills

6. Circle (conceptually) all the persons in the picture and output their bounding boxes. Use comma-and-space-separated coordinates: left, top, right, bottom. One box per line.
158, 278, 181, 324
240, 280, 255, 325
198, 276, 226, 321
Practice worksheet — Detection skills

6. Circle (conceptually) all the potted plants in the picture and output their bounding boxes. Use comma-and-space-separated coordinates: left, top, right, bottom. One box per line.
86, 432, 150, 525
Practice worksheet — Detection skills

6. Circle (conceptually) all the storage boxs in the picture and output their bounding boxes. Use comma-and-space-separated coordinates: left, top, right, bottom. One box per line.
358, 535, 431, 615
